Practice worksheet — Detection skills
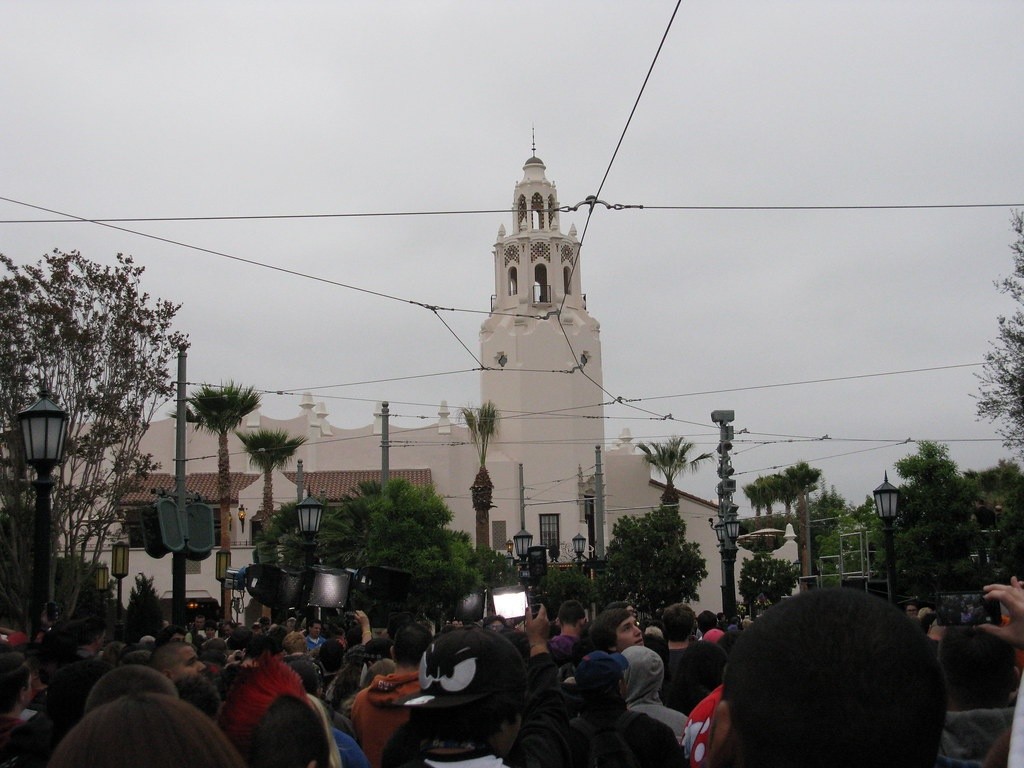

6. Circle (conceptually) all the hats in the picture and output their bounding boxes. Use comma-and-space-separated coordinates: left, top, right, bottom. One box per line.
390, 625, 529, 705
362, 637, 393, 663
576, 651, 632, 683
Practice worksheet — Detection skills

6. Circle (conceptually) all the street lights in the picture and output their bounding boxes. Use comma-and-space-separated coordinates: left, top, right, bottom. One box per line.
568, 533, 588, 563
110, 539, 130, 640
871, 471, 902, 608
94, 564, 109, 635
511, 522, 533, 571
707, 512, 742, 624
294, 491, 326, 624
16, 376, 70, 644
216, 548, 232, 627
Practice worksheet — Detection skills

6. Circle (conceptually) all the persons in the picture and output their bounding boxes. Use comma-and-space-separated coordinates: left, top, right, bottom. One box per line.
0, 574, 1021, 767
